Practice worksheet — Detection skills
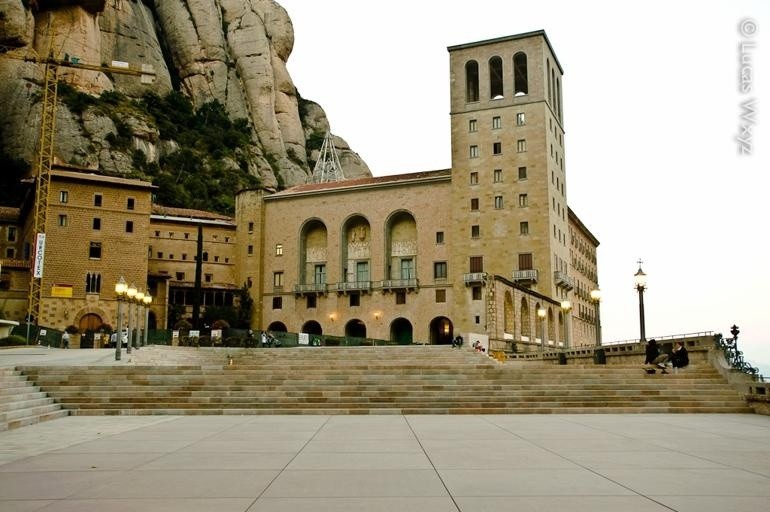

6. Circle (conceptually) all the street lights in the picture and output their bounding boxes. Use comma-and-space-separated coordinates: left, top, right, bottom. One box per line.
538, 308, 547, 352
633, 258, 647, 345
115, 275, 153, 360
731, 324, 740, 356
591, 288, 604, 346
561, 301, 573, 349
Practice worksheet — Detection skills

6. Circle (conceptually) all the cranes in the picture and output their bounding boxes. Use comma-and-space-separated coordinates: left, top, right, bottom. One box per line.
3, 47, 158, 325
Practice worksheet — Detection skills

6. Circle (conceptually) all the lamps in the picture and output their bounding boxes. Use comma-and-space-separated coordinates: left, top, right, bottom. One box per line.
330, 315, 334, 322
375, 313, 379, 320
64, 309, 69, 320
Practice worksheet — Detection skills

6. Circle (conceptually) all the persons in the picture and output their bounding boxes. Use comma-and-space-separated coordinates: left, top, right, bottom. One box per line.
473, 340, 480, 348
475, 344, 486, 353
61, 330, 70, 347
645, 339, 669, 368
102, 325, 129, 348
261, 330, 267, 347
248, 328, 255, 346
452, 332, 463, 349
658, 341, 690, 374
268, 330, 274, 346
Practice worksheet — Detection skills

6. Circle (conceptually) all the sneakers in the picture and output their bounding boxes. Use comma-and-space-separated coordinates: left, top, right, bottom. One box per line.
658, 361, 673, 368
